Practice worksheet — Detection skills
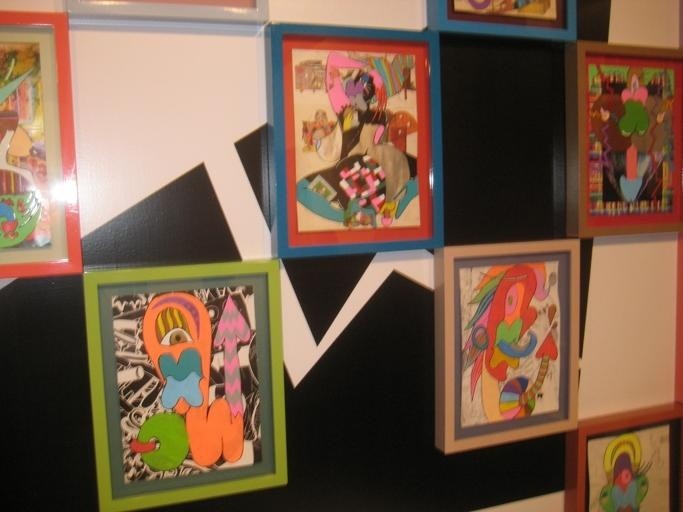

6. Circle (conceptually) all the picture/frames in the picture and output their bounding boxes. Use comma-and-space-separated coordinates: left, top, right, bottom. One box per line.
433, 238, 581, 455
65, 3, 269, 36
264, 22, 444, 260
80, 258, 288, 512
427, 0, 578, 45
563, 400, 681, 511
564, 40, 681, 238
2, 10, 86, 277
